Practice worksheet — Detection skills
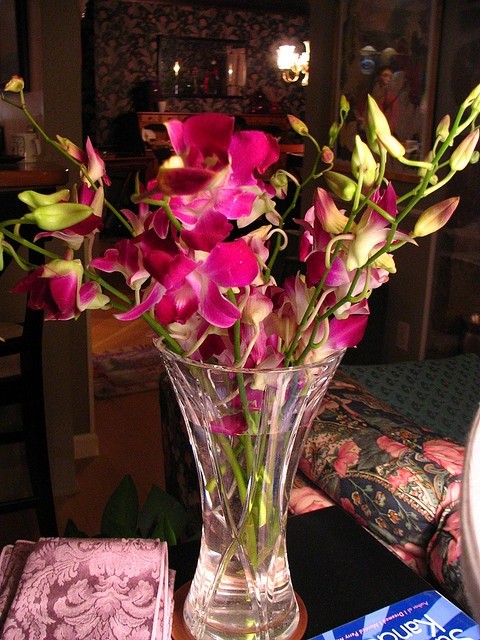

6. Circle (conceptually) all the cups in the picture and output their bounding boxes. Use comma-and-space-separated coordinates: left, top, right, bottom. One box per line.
158, 100, 168, 112
11, 132, 43, 164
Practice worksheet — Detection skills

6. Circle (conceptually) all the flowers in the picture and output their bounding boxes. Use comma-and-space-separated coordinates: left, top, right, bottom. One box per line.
0, 64, 480, 640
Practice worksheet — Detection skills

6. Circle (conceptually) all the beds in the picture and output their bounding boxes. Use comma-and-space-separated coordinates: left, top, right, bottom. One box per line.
296, 352, 480, 621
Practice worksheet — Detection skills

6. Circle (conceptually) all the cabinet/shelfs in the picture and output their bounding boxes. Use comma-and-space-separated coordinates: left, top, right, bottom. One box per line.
137, 110, 300, 173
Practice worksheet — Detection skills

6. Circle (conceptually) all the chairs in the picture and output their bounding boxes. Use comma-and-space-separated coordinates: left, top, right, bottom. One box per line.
1, 206, 60, 540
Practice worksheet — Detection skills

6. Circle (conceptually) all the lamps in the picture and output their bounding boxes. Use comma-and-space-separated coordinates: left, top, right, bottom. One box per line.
275, 39, 310, 89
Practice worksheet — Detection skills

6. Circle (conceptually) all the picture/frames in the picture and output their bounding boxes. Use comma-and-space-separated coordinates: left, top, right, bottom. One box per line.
156, 34, 248, 99
326, 0, 442, 184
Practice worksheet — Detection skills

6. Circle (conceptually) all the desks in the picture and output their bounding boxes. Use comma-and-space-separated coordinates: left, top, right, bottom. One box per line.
166, 505, 477, 640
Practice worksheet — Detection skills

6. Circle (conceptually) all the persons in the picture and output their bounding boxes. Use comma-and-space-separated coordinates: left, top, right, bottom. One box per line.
187, 64, 202, 95
368, 65, 397, 115
208, 64, 225, 94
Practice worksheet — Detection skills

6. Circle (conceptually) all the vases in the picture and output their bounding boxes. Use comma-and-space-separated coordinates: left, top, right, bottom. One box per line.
150, 334, 349, 640
